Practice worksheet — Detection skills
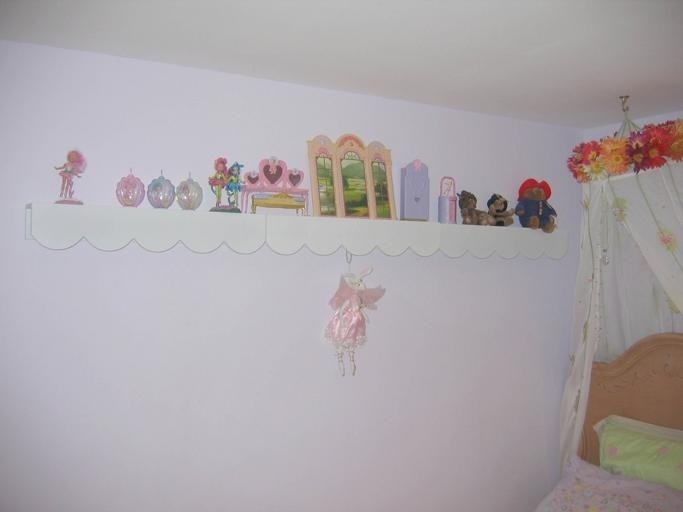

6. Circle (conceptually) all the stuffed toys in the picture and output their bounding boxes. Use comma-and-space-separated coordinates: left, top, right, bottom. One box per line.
454, 191, 515, 226
515, 177, 557, 233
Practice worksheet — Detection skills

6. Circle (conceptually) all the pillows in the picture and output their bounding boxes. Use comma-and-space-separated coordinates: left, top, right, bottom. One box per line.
534, 416, 683, 512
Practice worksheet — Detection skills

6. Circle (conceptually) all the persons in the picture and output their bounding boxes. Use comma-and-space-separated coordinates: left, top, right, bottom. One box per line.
53, 150, 84, 195
325, 271, 384, 378
207, 157, 242, 211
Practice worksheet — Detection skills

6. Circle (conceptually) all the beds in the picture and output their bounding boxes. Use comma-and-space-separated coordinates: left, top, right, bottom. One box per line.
534, 329, 683, 512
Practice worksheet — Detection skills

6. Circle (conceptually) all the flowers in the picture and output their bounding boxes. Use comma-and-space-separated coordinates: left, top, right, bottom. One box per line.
565, 119, 682, 184
